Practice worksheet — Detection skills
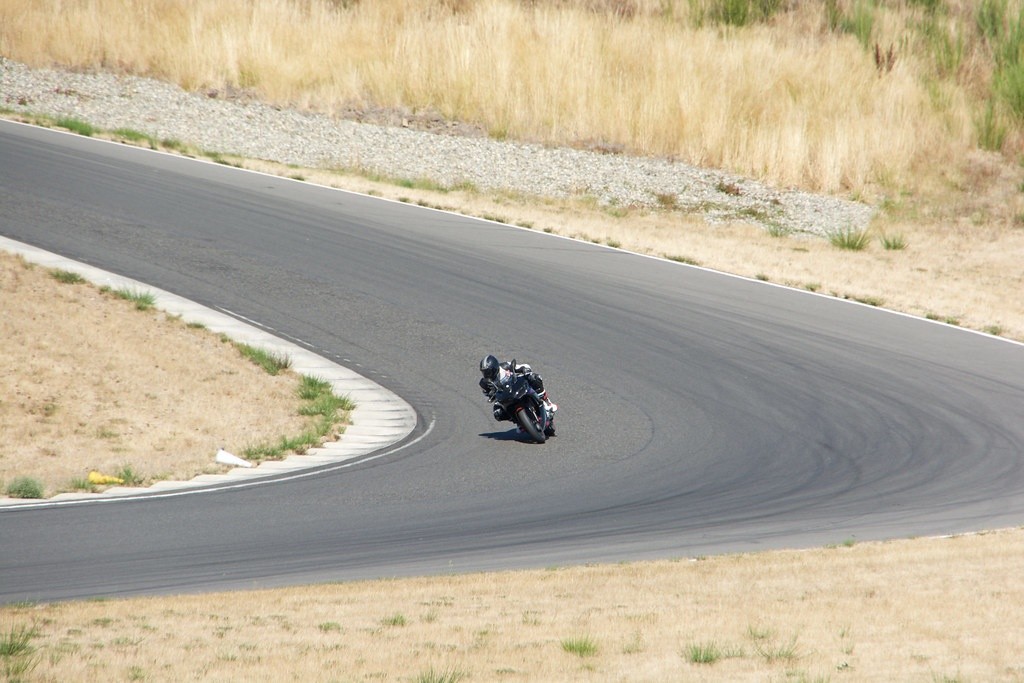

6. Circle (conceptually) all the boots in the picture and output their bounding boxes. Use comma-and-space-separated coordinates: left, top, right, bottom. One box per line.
537, 389, 558, 412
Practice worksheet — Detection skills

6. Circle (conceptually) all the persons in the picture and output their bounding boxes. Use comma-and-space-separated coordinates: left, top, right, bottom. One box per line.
479, 355, 557, 433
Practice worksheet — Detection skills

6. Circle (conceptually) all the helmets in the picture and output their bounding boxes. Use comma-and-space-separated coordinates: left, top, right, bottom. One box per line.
480, 355, 499, 380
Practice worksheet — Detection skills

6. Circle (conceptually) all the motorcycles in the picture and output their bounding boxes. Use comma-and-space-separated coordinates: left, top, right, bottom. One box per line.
482, 357, 556, 445
